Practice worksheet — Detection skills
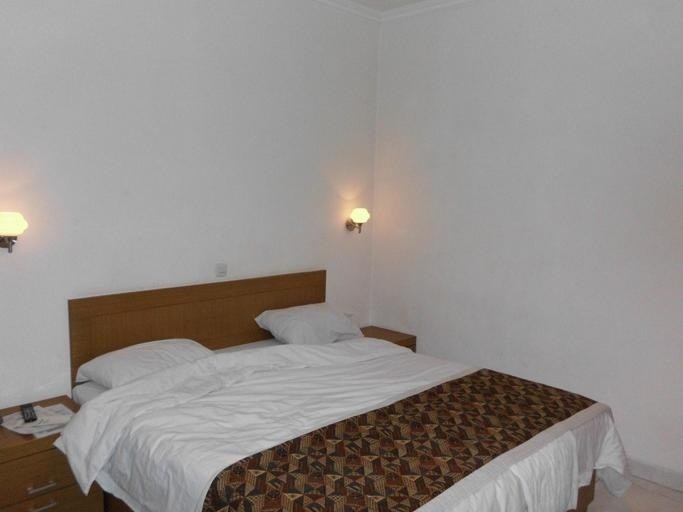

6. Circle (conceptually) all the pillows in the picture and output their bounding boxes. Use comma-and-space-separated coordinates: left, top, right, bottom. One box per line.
251, 303, 362, 346
75, 338, 214, 387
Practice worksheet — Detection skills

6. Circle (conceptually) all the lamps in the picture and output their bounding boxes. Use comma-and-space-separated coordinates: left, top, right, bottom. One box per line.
1, 210, 29, 252
345, 206, 370, 234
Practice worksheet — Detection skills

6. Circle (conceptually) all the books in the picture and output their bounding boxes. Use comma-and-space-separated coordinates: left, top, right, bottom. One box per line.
29, 403, 75, 439
1, 405, 70, 436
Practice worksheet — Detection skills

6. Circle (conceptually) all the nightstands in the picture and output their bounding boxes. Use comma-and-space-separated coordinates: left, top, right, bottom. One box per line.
1, 392, 105, 511
354, 324, 416, 354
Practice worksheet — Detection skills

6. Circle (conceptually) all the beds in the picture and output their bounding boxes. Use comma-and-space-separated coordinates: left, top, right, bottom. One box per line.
67, 268, 613, 512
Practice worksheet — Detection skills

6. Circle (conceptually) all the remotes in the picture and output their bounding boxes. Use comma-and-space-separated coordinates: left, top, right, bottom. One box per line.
19, 403, 37, 422
